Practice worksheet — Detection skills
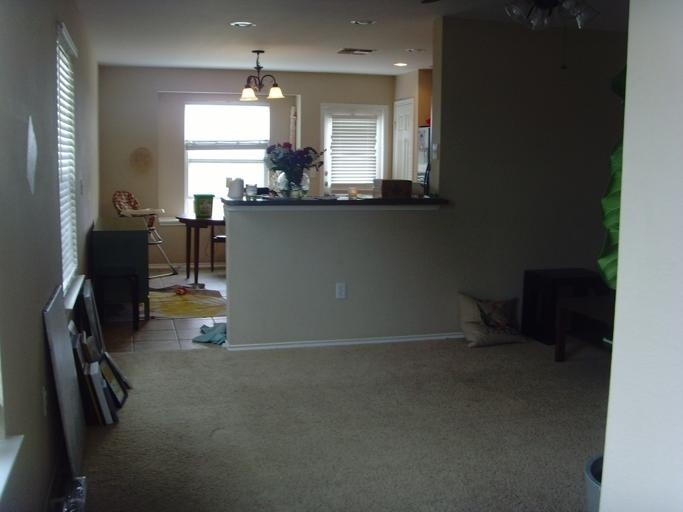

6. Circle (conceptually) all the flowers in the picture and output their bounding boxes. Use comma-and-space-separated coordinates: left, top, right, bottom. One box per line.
263, 141, 329, 187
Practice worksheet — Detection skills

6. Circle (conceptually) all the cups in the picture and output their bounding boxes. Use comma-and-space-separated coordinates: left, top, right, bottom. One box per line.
348, 186, 357, 201
245, 185, 258, 201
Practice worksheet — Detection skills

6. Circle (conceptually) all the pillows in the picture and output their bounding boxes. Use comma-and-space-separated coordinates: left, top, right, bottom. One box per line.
454, 289, 529, 348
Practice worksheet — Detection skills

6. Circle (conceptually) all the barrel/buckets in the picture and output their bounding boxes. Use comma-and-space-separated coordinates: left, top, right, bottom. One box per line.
193, 192, 214, 218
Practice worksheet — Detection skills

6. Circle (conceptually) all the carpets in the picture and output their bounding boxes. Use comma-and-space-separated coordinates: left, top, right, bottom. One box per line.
89, 274, 228, 322
56, 332, 611, 512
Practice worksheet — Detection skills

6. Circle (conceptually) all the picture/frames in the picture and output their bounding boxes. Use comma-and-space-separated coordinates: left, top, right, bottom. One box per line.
75, 331, 131, 430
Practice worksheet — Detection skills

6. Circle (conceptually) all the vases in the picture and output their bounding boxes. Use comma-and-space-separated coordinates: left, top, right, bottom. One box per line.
280, 180, 309, 200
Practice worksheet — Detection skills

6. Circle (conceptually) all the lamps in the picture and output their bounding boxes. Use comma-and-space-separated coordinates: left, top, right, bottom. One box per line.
238, 49, 286, 102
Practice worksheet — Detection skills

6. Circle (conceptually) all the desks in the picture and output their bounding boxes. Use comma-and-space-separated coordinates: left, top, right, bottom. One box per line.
91, 224, 156, 331
175, 215, 224, 285
